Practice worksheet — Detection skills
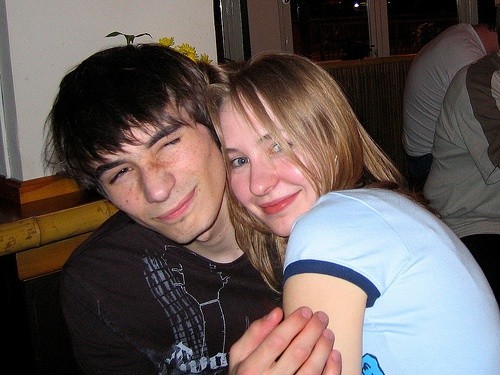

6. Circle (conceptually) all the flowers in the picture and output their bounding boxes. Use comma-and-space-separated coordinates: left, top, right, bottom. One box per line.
105, 31, 213, 65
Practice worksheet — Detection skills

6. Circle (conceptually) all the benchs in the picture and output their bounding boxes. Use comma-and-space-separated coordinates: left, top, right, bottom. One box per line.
313, 53, 416, 192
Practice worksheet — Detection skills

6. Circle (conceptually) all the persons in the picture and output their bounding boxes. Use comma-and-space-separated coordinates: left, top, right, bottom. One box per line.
423, 44, 500, 311
50, 41, 345, 375
402, 3, 500, 197
194, 50, 500, 375
